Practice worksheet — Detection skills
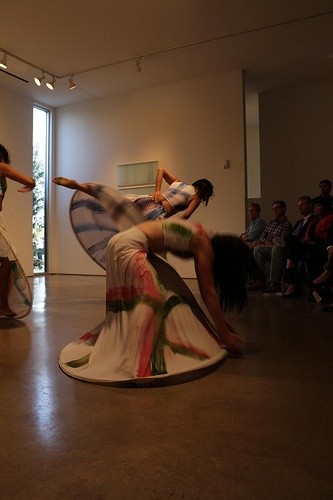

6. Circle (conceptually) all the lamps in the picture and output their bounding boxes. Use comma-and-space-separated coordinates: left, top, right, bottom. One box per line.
70, 74, 75, 89
32, 72, 48, 86
0, 53, 7, 69
45, 76, 58, 90
134, 56, 144, 72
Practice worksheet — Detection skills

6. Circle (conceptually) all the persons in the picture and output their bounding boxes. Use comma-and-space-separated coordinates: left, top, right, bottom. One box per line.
52, 167, 215, 260
64, 217, 263, 378
247, 196, 315, 293
0, 143, 35, 316
313, 180, 333, 198
280, 197, 333, 312
240, 201, 292, 284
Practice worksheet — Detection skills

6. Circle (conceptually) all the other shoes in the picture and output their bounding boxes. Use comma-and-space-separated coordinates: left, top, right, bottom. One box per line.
262, 282, 281, 293
281, 286, 300, 298
246, 280, 266, 290
302, 280, 330, 287
282, 273, 301, 285
320, 305, 333, 312
